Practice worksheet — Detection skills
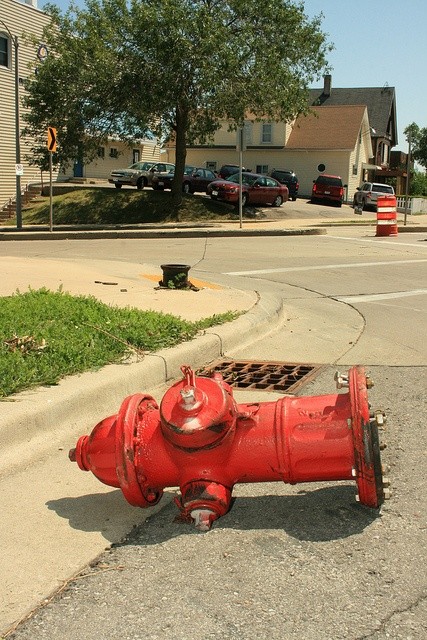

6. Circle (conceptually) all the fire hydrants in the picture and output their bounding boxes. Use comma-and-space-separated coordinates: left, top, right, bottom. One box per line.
69, 363, 392, 531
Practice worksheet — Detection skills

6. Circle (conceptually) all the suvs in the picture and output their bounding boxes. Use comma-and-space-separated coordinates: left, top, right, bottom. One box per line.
310, 174, 344, 207
353, 182, 395, 211
267, 168, 299, 201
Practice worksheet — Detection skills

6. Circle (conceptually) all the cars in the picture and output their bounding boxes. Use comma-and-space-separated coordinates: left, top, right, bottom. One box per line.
152, 166, 224, 195
214, 164, 246, 181
207, 172, 289, 207
108, 160, 176, 190
